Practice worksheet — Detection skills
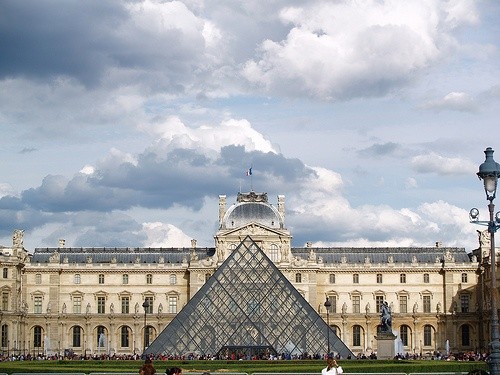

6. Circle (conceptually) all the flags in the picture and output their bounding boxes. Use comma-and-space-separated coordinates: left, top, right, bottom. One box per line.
246, 168, 252, 175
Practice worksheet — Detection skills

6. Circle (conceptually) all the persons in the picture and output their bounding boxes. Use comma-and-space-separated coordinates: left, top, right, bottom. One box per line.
0, 350, 487, 364
321, 357, 343, 375
138, 362, 182, 375
381, 302, 391, 331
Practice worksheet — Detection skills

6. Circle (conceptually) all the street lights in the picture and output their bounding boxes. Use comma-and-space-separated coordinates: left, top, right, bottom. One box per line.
323, 297, 332, 358
142, 298, 149, 360
469, 147, 500, 375
